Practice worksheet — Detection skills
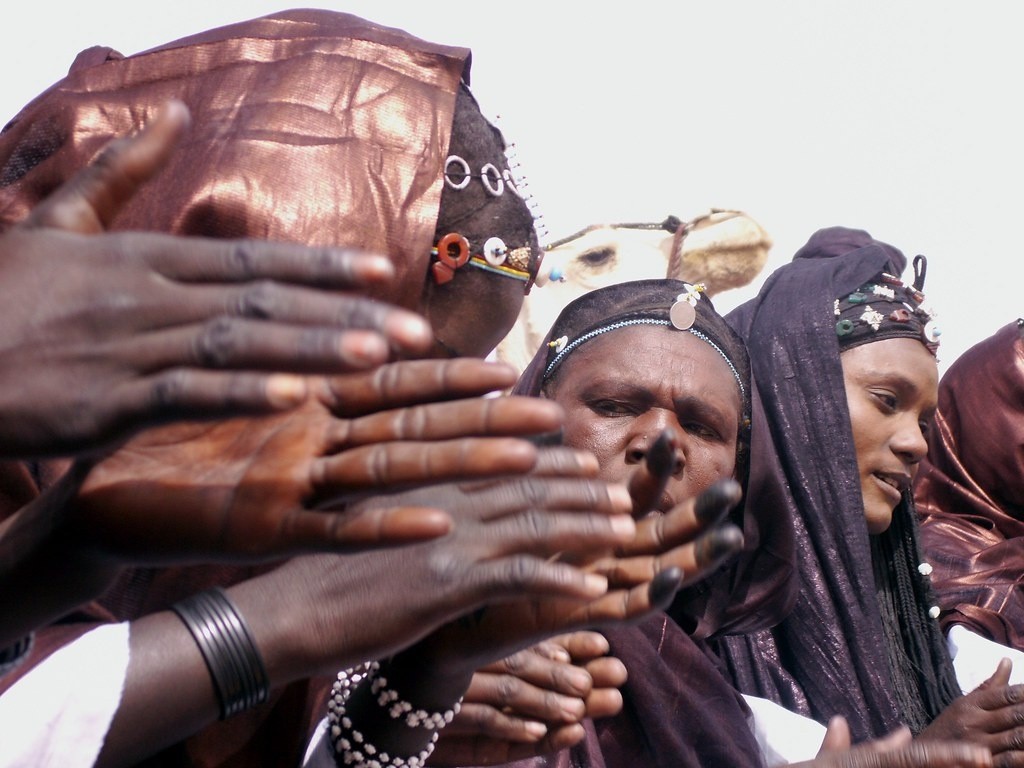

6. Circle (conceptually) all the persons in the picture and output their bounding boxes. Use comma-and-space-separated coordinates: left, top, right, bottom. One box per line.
1, 8, 1024, 768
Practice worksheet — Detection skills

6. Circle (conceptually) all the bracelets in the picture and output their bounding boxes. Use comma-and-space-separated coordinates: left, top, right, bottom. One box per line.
326, 662, 438, 768
169, 588, 271, 722
371, 660, 465, 729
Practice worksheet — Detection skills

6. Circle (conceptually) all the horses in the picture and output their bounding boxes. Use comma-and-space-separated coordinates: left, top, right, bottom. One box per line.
483, 204, 772, 402
